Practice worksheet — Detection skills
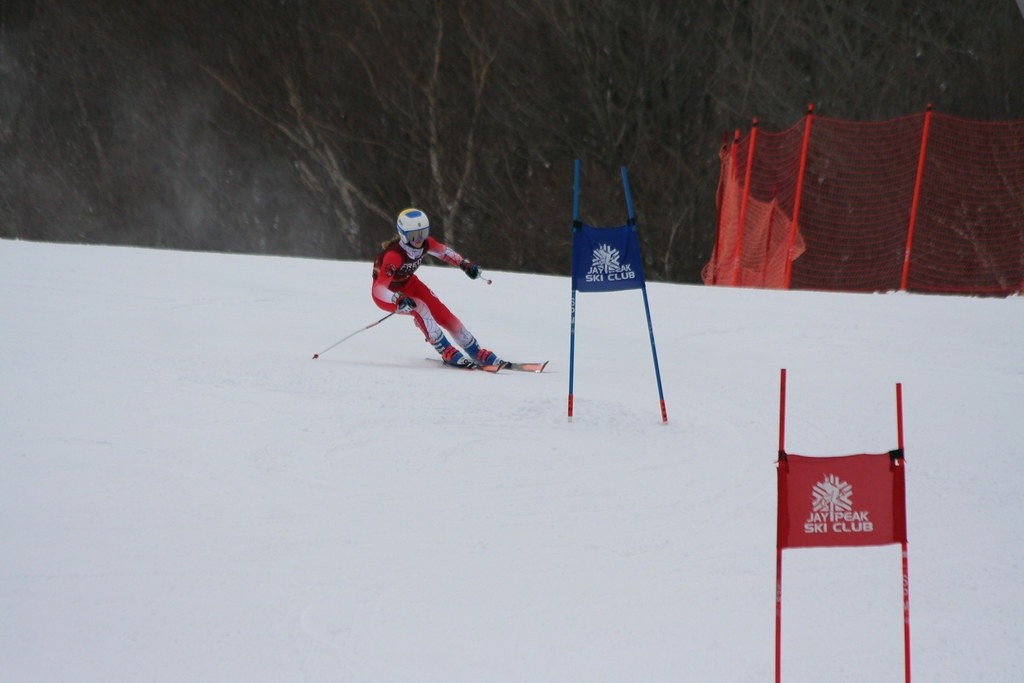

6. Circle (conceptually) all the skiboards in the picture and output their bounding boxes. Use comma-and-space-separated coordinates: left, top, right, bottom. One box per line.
424, 356, 550, 374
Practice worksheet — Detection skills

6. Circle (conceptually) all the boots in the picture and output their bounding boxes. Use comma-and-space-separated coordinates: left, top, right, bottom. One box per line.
434, 335, 466, 365
464, 337, 500, 366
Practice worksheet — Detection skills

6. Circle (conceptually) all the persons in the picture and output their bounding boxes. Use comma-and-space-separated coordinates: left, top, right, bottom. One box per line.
371, 208, 502, 370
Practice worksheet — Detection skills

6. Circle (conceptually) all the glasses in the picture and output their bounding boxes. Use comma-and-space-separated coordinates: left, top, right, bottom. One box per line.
404, 225, 430, 241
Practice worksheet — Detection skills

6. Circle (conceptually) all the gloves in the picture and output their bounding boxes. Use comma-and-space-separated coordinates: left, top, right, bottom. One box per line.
396, 297, 417, 313
459, 258, 479, 280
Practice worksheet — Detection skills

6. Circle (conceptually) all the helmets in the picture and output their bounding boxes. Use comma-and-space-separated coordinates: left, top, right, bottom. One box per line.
398, 208, 430, 244
